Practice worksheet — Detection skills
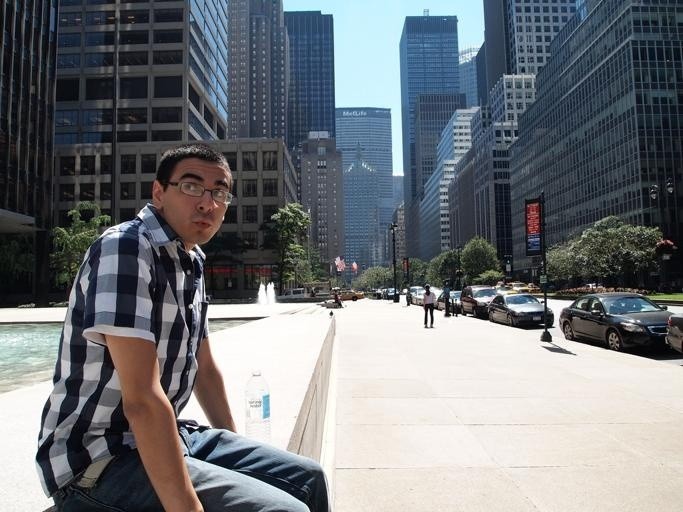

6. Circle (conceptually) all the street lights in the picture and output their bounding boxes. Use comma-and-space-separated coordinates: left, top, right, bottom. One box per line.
389, 222, 399, 303
649, 178, 675, 240
455, 246, 462, 286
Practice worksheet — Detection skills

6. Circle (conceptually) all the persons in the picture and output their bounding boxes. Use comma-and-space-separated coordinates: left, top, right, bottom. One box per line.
335, 290, 344, 308
35, 143, 329, 512
443, 287, 451, 316
423, 284, 436, 328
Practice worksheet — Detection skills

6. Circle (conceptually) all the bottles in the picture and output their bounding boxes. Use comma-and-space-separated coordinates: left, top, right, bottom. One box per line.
245, 368, 270, 444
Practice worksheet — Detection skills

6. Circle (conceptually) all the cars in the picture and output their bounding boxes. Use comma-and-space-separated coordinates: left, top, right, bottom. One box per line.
559, 282, 683, 354
329, 281, 554, 328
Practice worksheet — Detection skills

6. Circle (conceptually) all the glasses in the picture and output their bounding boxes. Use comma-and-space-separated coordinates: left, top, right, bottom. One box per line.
162, 181, 234, 205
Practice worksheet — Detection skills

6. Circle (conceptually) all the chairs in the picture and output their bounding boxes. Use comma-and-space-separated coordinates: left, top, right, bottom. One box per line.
581, 303, 602, 310
